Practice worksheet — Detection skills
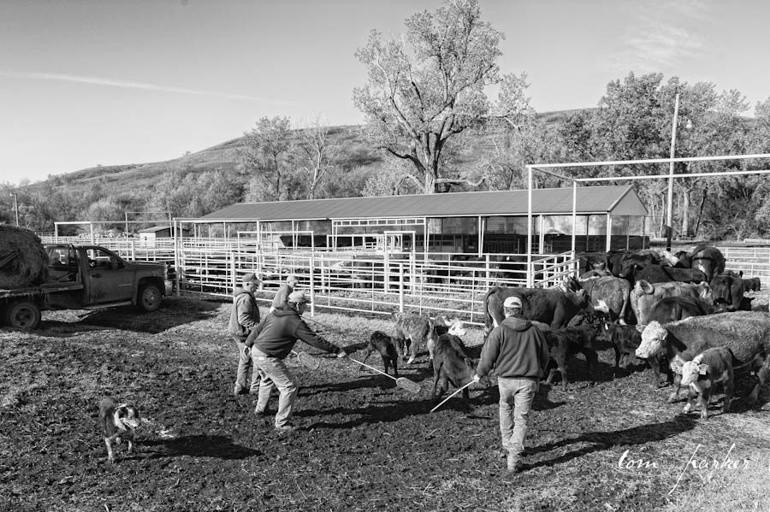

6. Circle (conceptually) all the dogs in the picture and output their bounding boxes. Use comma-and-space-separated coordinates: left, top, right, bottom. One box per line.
98, 396, 142, 464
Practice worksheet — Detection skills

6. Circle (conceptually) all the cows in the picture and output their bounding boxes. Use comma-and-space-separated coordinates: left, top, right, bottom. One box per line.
358, 330, 399, 377
431, 333, 481, 400
428, 315, 468, 359
389, 311, 433, 365
118, 242, 483, 295
483, 245, 770, 420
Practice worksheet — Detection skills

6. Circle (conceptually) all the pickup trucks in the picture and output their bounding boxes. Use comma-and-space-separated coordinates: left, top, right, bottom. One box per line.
0, 244, 169, 331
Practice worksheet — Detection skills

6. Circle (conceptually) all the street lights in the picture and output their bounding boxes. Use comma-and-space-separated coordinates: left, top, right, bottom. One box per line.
665, 115, 692, 252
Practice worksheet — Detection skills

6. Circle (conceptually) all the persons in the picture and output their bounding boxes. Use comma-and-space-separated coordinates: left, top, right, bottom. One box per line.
473, 296, 550, 472
242, 292, 346, 430
228, 273, 261, 395
269, 275, 299, 313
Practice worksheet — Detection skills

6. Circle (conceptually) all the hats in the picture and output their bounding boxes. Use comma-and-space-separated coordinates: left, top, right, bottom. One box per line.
288, 292, 311, 304
243, 273, 262, 284
504, 297, 522, 308
